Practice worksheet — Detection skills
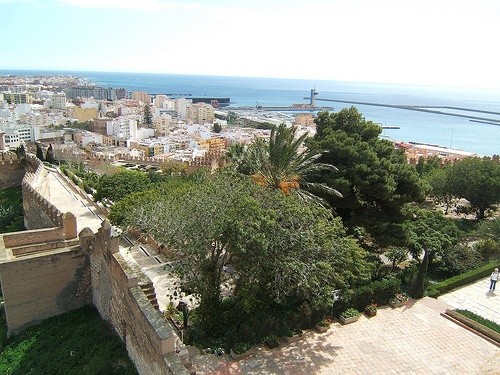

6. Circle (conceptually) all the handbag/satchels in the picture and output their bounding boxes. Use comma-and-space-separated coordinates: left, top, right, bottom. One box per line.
497, 273, 500, 281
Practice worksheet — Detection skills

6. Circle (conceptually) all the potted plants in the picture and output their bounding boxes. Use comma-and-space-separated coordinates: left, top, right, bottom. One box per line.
230, 342, 252, 360
284, 329, 306, 343
263, 335, 280, 348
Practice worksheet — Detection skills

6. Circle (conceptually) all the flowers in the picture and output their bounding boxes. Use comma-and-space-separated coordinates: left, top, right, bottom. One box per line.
319, 318, 331, 326
364, 303, 377, 312
390, 292, 408, 304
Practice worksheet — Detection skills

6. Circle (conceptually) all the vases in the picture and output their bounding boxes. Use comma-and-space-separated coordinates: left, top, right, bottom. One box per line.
389, 293, 410, 307
365, 309, 376, 317
340, 308, 359, 325
316, 323, 329, 332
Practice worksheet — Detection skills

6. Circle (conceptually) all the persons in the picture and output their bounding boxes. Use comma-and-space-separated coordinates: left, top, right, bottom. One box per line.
489, 268, 499, 293
